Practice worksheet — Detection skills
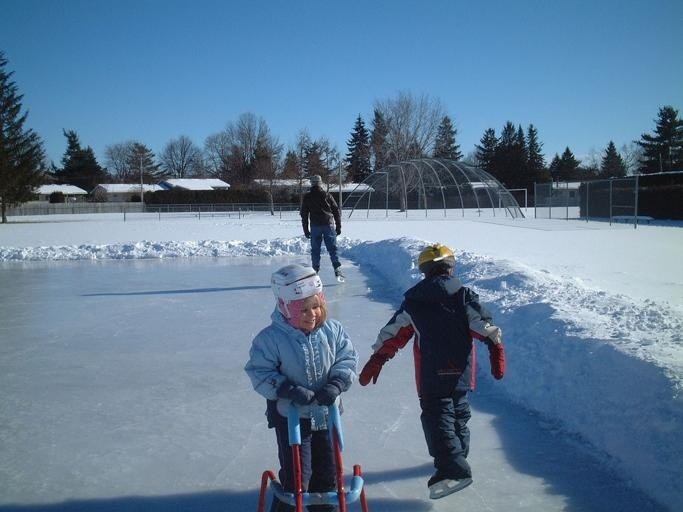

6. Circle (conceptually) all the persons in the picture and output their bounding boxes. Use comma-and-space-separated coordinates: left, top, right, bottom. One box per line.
298, 173, 345, 281
243, 261, 359, 512
358, 242, 508, 487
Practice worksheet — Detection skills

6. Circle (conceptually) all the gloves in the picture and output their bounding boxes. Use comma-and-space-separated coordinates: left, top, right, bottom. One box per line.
490, 343, 505, 379
305, 232, 311, 238
277, 382, 315, 405
317, 378, 345, 405
359, 354, 387, 385
336, 230, 341, 235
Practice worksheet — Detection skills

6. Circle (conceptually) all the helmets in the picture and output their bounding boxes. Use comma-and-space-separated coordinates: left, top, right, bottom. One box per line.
311, 175, 322, 183
419, 243, 453, 267
272, 264, 324, 303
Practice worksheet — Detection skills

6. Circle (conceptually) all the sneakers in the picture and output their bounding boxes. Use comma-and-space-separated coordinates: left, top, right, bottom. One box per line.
335, 271, 345, 277
427, 470, 443, 488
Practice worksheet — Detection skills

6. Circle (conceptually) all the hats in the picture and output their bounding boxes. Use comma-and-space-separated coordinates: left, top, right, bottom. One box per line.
279, 292, 325, 328
